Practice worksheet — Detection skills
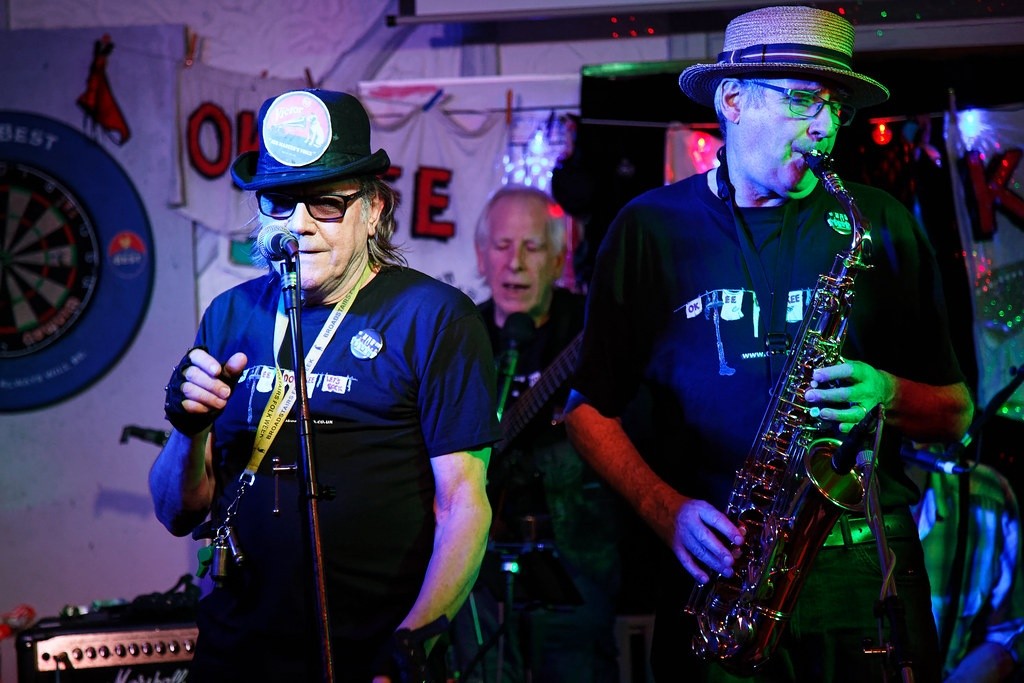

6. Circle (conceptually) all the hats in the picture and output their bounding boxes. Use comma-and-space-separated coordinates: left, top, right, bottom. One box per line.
680, 7, 891, 108
230, 89, 390, 190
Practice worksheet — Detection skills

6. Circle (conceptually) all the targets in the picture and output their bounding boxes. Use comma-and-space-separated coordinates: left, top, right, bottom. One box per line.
0, 156, 100, 359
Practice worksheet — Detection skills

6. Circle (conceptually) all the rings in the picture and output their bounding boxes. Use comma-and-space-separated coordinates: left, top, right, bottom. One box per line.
858, 402, 869, 416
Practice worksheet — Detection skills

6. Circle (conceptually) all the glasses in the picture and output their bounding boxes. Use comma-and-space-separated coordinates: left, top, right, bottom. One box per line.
256, 191, 363, 222
747, 78, 855, 127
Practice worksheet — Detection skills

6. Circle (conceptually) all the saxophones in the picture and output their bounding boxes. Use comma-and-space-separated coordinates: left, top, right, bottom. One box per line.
680, 147, 884, 676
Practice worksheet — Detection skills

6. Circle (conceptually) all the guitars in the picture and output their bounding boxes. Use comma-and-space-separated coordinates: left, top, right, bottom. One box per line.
494, 331, 594, 461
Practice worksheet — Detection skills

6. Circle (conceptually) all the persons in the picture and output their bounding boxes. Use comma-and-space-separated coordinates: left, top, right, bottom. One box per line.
563, 9, 978, 681
450, 180, 626, 683
145, 86, 511, 683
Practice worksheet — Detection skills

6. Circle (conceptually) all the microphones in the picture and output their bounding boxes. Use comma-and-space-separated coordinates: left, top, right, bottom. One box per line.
830, 402, 886, 475
495, 310, 537, 423
888, 441, 965, 474
256, 223, 299, 261
